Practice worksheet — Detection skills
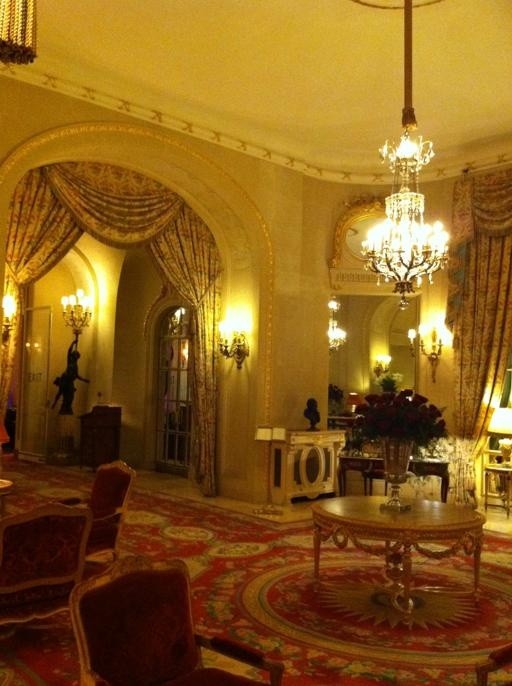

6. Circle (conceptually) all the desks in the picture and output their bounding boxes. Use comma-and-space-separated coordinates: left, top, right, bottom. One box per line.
269, 430, 346, 500
337, 455, 450, 504
312, 495, 487, 624
483, 464, 512, 519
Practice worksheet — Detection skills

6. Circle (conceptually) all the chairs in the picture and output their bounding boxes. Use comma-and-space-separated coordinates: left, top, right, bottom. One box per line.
70, 555, 284, 686
0, 501, 96, 648
87, 459, 136, 563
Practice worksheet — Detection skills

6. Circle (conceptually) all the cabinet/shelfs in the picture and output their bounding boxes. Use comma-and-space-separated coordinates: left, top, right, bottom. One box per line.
77, 405, 122, 466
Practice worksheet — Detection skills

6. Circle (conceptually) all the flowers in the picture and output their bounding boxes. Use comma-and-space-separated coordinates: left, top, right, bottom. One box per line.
342, 390, 448, 449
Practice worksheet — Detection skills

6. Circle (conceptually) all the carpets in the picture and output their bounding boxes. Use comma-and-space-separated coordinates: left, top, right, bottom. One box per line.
1, 459, 512, 685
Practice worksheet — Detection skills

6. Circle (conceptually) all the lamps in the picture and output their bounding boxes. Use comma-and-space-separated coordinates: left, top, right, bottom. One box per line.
60, 290, 93, 333
374, 352, 391, 375
405, 314, 455, 383
2, 294, 17, 340
359, 1, 450, 309
346, 393, 361, 413
487, 406, 512, 469
218, 307, 252, 370
327, 298, 347, 351
477, 643, 512, 686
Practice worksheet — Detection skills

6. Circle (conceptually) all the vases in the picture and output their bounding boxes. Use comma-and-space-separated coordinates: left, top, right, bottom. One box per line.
378, 437, 414, 512
